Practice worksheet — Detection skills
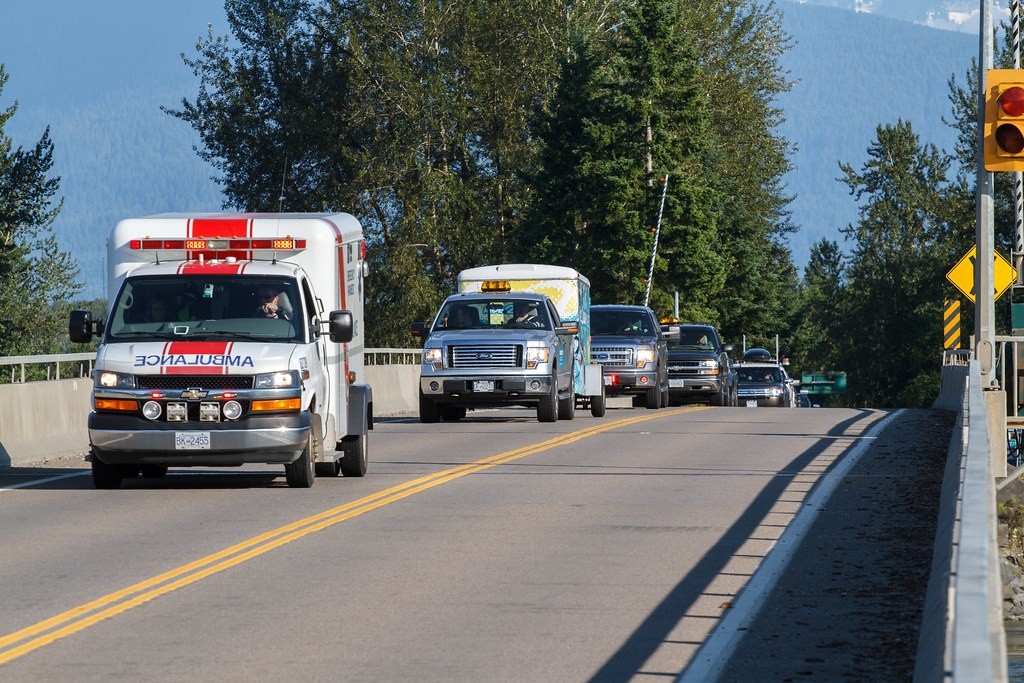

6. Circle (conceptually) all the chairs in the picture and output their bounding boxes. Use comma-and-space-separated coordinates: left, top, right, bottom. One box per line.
512, 305, 546, 327
276, 290, 293, 315
455, 306, 480, 328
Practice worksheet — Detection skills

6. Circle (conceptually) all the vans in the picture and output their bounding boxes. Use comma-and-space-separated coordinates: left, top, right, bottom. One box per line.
456, 264, 606, 417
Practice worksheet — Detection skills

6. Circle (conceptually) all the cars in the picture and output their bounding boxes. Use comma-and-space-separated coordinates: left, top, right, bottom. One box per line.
727, 348, 790, 407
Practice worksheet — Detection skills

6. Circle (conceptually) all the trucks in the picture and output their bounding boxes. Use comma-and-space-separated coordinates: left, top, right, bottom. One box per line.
67, 211, 375, 489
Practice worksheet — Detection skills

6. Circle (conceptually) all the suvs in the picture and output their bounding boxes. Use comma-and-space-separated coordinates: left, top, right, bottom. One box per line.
410, 290, 579, 425
589, 305, 670, 411
658, 319, 738, 407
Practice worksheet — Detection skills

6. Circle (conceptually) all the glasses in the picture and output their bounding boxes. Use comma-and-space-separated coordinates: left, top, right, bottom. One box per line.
259, 295, 274, 300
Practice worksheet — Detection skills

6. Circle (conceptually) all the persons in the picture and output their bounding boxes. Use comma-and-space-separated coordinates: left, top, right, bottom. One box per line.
696, 331, 713, 347
615, 315, 643, 335
506, 304, 541, 328
150, 302, 168, 322
449, 305, 475, 327
247, 284, 293, 322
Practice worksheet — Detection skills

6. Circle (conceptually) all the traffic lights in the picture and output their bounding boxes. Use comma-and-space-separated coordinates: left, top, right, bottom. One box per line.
983, 67, 1023, 173
